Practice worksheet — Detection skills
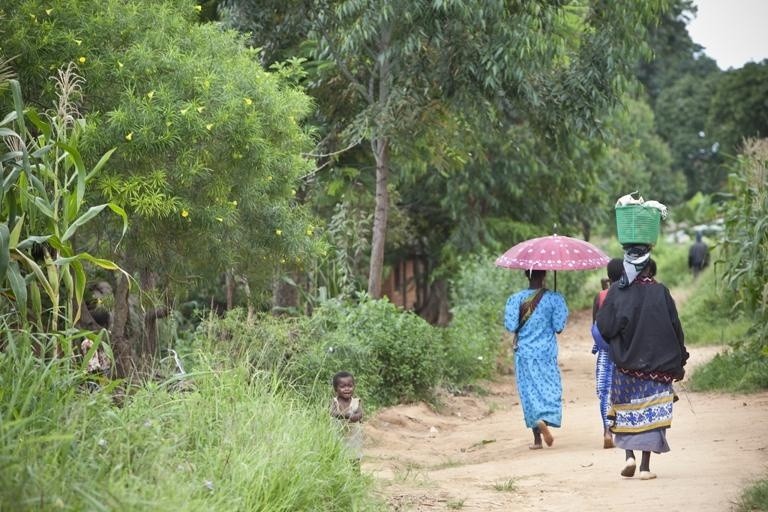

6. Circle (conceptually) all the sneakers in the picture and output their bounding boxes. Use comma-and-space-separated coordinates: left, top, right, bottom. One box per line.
639, 470, 657, 481
619, 457, 637, 477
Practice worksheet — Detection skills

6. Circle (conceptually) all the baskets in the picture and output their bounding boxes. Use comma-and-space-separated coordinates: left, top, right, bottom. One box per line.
614, 205, 661, 248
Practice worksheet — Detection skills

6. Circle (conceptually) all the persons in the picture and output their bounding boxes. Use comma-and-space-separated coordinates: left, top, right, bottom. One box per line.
504, 270, 570, 449
329, 370, 363, 476
81, 307, 124, 415
688, 231, 710, 275
591, 243, 691, 483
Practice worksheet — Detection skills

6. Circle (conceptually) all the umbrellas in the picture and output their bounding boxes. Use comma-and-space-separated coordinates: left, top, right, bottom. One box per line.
494, 223, 610, 292
